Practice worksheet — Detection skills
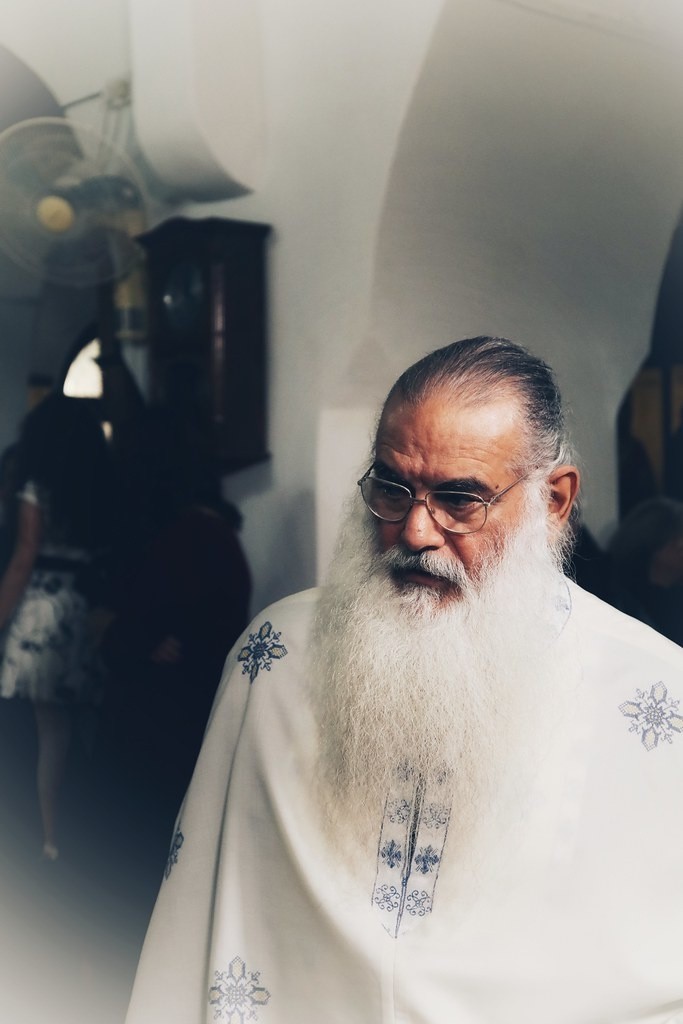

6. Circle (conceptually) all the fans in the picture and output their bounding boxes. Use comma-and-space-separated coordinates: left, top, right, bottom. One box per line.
0, 117, 152, 287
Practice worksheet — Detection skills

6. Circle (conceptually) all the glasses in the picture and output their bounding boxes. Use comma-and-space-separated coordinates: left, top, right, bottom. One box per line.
357, 463, 543, 534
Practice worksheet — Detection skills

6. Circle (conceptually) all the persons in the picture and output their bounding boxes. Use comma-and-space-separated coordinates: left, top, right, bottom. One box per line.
1, 322, 683, 1024
125, 336, 683, 1024
